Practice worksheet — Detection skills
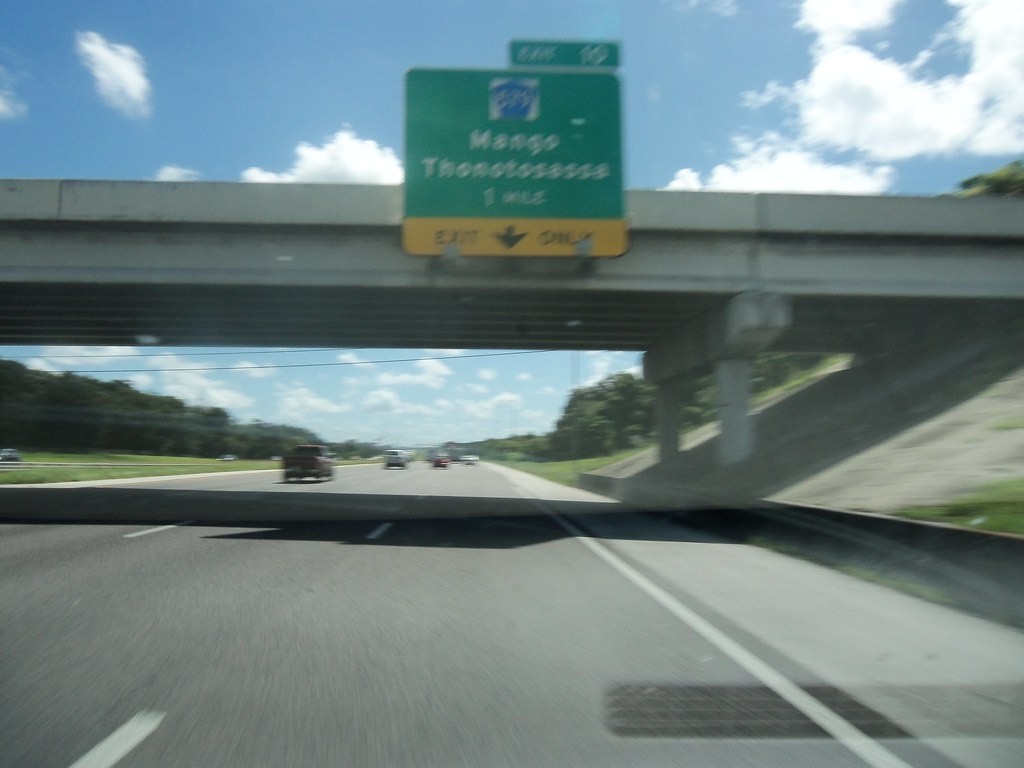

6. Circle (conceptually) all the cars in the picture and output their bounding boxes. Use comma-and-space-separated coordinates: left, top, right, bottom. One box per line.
0, 449, 22, 463
384, 449, 406, 468
284, 444, 333, 480
